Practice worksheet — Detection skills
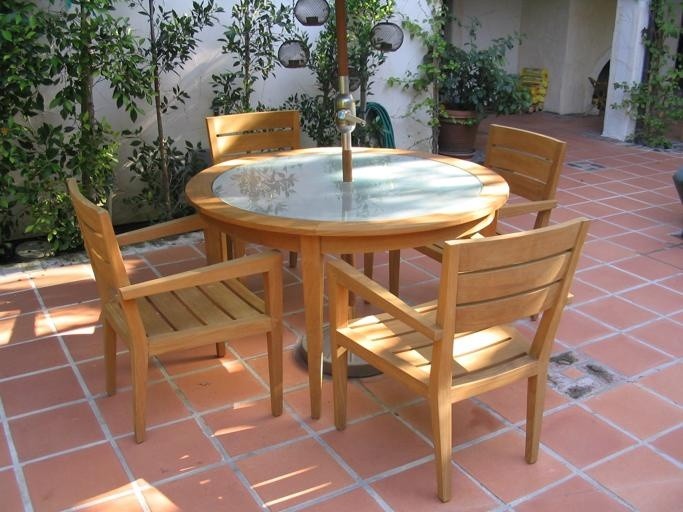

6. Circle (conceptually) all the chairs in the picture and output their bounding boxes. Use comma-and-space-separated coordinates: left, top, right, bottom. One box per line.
64, 176, 285, 445
327, 216, 588, 500
390, 122, 568, 304
206, 109, 300, 281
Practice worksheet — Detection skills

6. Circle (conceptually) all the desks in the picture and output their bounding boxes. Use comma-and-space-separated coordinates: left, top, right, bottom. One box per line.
184, 145, 514, 421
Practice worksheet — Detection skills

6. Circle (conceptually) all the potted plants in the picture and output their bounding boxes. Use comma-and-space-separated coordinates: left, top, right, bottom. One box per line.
388, 1, 537, 161
